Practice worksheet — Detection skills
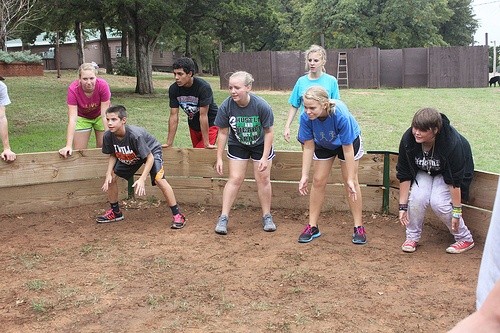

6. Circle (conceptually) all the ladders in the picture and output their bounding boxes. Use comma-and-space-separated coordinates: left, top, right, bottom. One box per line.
336, 51, 349, 90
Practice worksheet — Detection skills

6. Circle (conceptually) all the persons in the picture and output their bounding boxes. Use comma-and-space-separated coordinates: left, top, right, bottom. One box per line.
396, 107, 475, 254
444, 176, 500, 333
0, 76, 16, 162
214, 70, 276, 235
59, 62, 111, 159
297, 86, 367, 244
162, 56, 221, 149
284, 44, 340, 150
95, 105, 186, 229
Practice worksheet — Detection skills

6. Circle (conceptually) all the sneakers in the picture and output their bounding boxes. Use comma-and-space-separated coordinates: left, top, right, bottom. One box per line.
215, 216, 228, 235
445, 241, 475, 254
171, 213, 187, 229
262, 214, 277, 231
352, 225, 367, 244
401, 240, 418, 252
298, 224, 321, 243
96, 208, 125, 223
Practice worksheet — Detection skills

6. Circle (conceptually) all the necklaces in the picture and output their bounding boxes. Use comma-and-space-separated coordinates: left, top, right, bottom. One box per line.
421, 142, 435, 175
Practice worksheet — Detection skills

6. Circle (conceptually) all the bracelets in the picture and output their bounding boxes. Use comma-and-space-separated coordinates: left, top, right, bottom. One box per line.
399, 204, 408, 211
453, 207, 462, 219
